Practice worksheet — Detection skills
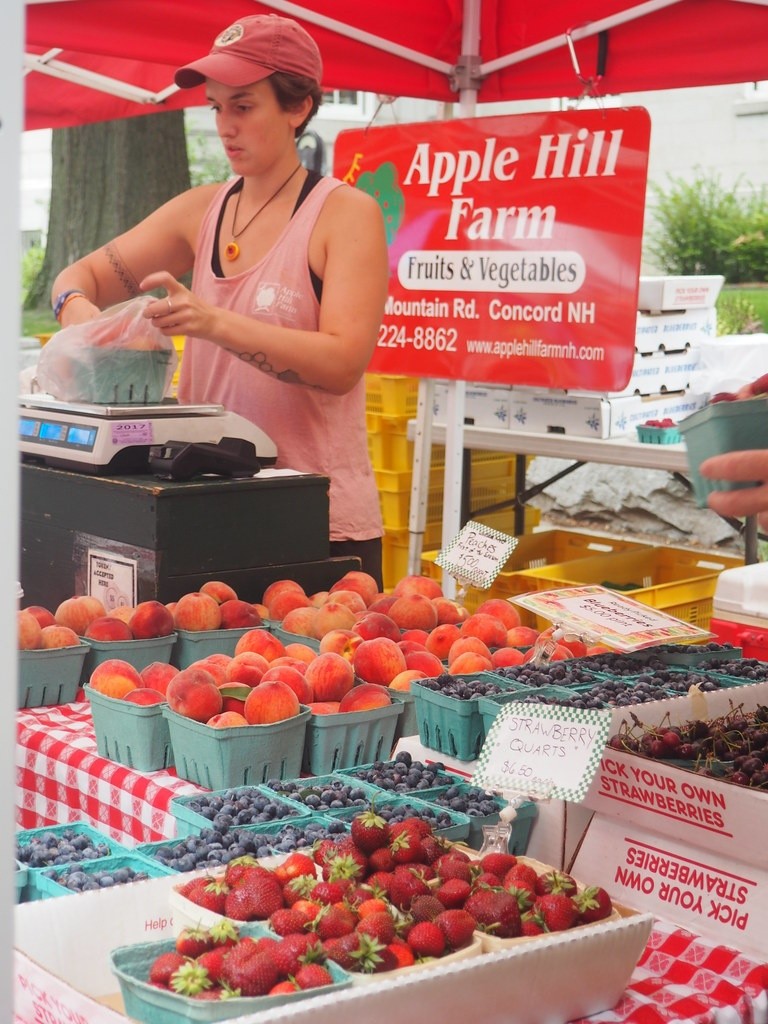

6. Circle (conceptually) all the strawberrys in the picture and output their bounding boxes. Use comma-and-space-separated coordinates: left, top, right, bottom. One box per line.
147, 812, 611, 1002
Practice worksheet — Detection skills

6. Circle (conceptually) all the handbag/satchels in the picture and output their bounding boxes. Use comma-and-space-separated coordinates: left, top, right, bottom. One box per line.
39, 294, 179, 405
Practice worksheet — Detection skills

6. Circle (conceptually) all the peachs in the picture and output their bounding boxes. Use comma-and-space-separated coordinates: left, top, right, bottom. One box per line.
18, 572, 611, 728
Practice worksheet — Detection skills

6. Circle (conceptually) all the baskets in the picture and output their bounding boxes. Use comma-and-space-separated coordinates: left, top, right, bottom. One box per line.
637, 422, 679, 445
18, 618, 768, 1024
69, 347, 171, 403
681, 399, 767, 506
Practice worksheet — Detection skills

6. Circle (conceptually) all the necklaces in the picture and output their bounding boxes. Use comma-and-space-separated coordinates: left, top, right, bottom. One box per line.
223, 159, 304, 264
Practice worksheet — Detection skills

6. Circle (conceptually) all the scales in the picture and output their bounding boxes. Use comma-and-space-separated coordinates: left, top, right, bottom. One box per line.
16, 392, 280, 478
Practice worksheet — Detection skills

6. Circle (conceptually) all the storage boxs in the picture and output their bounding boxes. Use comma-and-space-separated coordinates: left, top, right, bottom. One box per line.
16, 275, 766, 1024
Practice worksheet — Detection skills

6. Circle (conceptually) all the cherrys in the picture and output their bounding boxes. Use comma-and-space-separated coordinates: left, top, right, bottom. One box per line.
607, 699, 768, 796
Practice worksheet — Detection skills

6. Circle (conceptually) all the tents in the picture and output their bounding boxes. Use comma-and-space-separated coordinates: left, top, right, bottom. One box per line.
24, 0, 767, 598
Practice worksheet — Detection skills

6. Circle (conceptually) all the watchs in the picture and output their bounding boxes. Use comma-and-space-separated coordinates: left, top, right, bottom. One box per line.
51, 288, 87, 324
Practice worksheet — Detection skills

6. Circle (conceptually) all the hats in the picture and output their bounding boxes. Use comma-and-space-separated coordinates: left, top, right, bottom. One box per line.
174, 14, 322, 90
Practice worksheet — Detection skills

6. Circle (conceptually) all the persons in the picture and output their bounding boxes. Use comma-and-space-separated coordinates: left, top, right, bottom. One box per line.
49, 13, 387, 597
